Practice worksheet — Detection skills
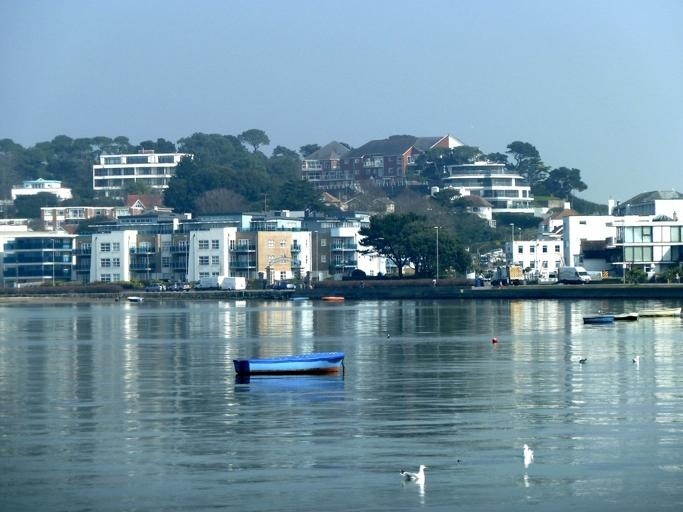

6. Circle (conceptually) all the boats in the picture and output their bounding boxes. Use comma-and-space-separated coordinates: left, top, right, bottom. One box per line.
288, 296, 309, 301
126, 296, 143, 301
233, 351, 344, 373
321, 296, 344, 302
583, 307, 683, 324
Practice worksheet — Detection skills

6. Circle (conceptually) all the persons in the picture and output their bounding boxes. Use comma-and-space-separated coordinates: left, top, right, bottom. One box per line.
431, 277, 436, 289
675, 272, 680, 283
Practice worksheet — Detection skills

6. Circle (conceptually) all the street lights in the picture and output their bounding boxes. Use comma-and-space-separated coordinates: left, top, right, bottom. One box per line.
433, 226, 438, 279
314, 231, 318, 283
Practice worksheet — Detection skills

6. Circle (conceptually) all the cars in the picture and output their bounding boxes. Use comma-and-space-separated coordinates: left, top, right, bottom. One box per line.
144, 282, 190, 292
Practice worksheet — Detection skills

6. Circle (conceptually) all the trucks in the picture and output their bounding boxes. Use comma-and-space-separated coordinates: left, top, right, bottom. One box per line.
490, 264, 591, 287
193, 276, 245, 291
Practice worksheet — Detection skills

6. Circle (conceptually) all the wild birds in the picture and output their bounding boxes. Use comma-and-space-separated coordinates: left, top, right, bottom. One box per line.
578, 357, 587, 363
633, 354, 639, 362
520, 442, 533, 460
399, 464, 427, 481
